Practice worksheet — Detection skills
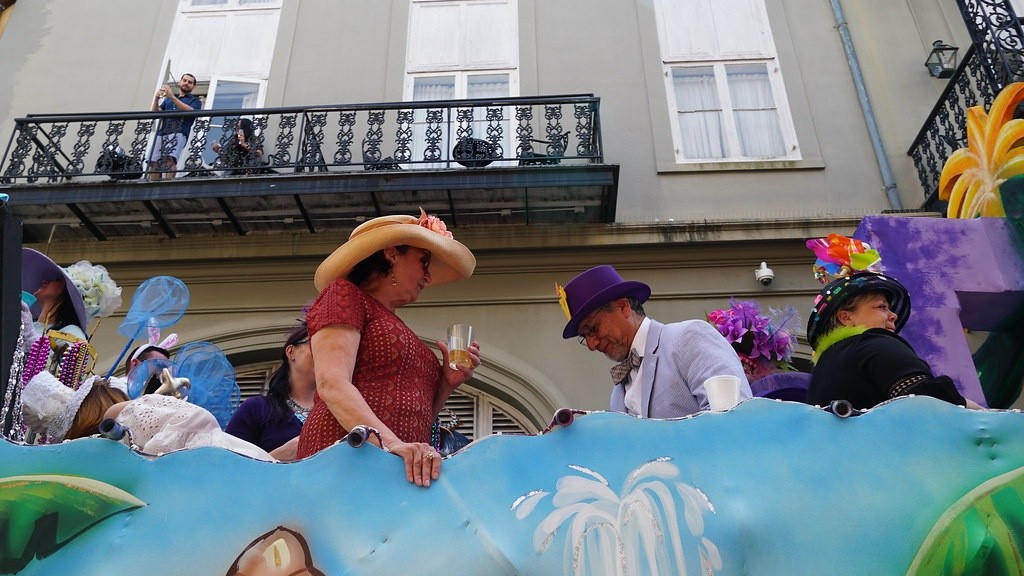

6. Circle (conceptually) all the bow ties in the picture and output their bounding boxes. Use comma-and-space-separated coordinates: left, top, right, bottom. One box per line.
610, 348, 640, 385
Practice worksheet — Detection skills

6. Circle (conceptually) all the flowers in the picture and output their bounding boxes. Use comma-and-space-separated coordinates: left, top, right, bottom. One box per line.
703, 298, 797, 362
414, 208, 456, 240
807, 234, 882, 281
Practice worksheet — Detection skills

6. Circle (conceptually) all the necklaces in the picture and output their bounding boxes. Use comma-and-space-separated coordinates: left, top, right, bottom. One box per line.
289, 396, 309, 411
23, 337, 89, 445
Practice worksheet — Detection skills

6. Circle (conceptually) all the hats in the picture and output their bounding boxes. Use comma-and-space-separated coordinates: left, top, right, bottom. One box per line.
20, 370, 102, 444
805, 232, 911, 352
703, 297, 813, 404
314, 206, 476, 292
21, 247, 122, 341
554, 265, 651, 339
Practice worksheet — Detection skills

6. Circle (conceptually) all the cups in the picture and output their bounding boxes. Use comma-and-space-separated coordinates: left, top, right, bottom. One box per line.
22, 291, 37, 309
447, 324, 472, 370
703, 375, 742, 411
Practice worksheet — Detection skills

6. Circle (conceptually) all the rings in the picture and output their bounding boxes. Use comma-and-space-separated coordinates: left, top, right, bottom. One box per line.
423, 451, 434, 459
471, 364, 479, 368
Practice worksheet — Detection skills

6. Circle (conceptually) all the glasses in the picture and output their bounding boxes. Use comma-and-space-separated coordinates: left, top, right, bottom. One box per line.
578, 300, 609, 346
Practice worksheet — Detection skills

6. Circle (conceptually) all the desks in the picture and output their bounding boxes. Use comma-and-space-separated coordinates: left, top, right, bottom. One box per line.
453, 140, 495, 167
96, 151, 143, 181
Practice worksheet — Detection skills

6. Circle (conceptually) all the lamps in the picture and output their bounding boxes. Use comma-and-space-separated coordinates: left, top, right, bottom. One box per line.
923, 38, 960, 78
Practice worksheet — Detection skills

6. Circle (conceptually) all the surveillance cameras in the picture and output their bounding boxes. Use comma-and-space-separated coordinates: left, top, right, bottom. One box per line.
757, 268, 775, 286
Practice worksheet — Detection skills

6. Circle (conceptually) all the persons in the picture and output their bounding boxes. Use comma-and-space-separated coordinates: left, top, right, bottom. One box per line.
295, 206, 481, 486
107, 318, 178, 394
21, 246, 122, 378
706, 297, 811, 403
23, 371, 275, 461
145, 74, 202, 179
555, 265, 753, 418
213, 118, 264, 175
806, 235, 984, 416
225, 299, 317, 461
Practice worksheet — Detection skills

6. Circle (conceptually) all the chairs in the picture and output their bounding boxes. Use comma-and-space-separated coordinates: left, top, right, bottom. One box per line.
362, 138, 401, 171
515, 130, 572, 167
